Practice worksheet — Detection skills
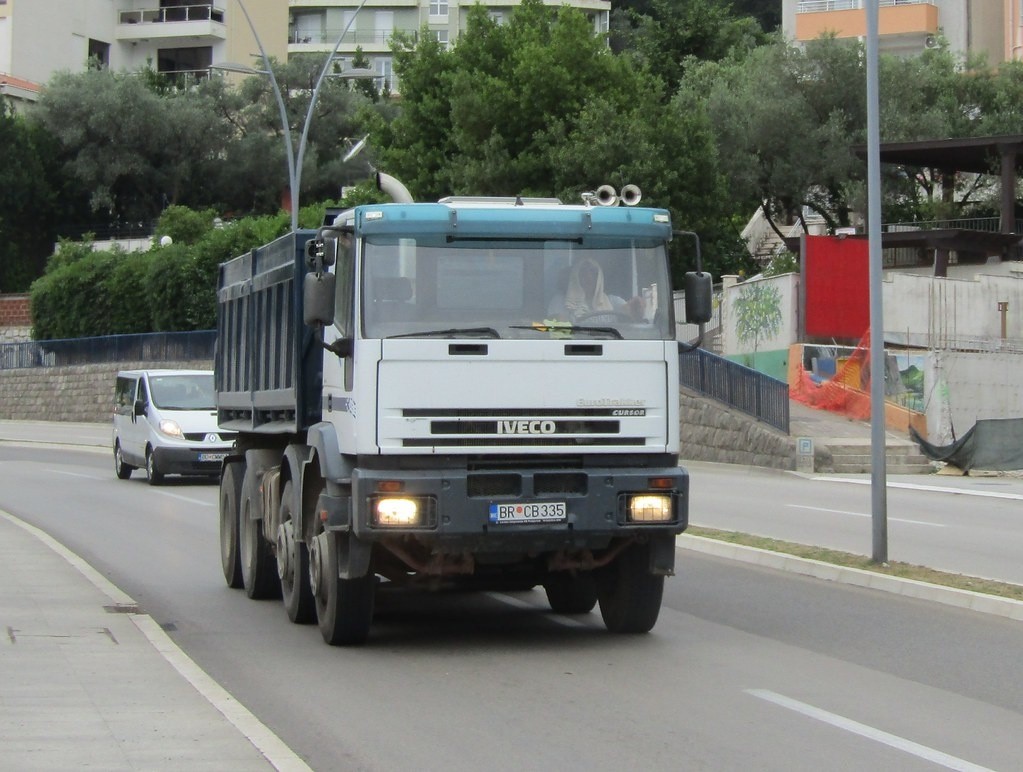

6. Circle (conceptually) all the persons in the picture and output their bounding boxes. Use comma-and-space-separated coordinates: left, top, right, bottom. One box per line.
547, 258, 647, 323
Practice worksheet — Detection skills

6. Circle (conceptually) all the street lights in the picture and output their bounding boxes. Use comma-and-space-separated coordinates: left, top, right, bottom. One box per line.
211, 64, 385, 232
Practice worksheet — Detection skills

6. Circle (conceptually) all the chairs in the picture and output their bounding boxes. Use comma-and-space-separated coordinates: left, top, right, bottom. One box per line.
545, 268, 571, 316
369, 276, 424, 323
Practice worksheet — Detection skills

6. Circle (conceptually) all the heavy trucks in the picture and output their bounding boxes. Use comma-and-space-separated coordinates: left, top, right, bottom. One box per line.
211, 172, 714, 647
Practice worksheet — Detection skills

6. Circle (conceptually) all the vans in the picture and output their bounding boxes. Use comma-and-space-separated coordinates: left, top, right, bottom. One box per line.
112, 371, 239, 486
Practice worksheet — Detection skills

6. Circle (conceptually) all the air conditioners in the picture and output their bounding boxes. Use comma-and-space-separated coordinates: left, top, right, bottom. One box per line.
925, 35, 942, 50
